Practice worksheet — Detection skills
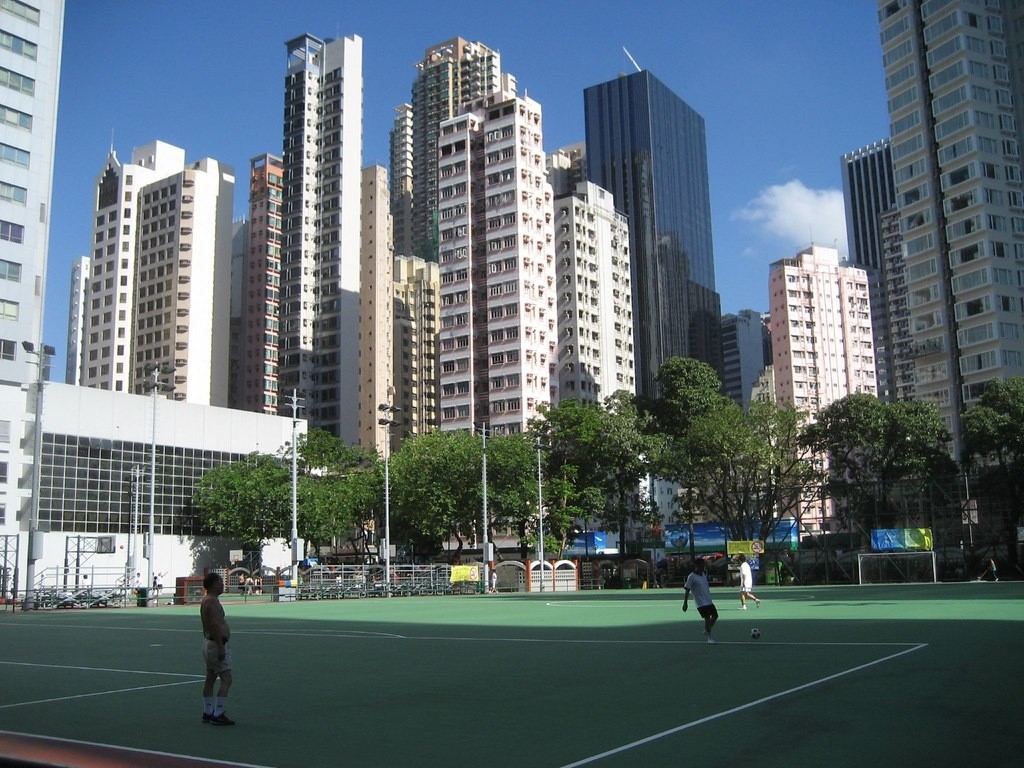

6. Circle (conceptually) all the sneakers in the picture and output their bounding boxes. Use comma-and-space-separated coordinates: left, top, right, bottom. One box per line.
209, 711, 235, 725
202, 709, 215, 722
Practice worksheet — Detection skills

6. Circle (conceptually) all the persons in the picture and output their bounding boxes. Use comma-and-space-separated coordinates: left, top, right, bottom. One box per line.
988, 559, 998, 581
153, 572, 169, 603
134, 573, 142, 594
118, 576, 130, 601
737, 554, 760, 610
238, 573, 262, 595
682, 558, 718, 643
299, 575, 305, 593
490, 570, 498, 593
336, 574, 342, 597
201, 573, 235, 724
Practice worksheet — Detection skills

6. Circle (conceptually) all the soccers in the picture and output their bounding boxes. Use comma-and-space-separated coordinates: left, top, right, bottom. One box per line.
750, 629, 761, 640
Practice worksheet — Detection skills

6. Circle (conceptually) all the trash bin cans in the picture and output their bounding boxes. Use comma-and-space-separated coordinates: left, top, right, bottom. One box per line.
136, 588, 152, 606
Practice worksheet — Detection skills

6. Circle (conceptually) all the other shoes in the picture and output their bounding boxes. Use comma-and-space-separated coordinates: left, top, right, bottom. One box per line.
737, 607, 746, 610
703, 631, 711, 635
707, 635, 716, 645
756, 598, 760, 608
